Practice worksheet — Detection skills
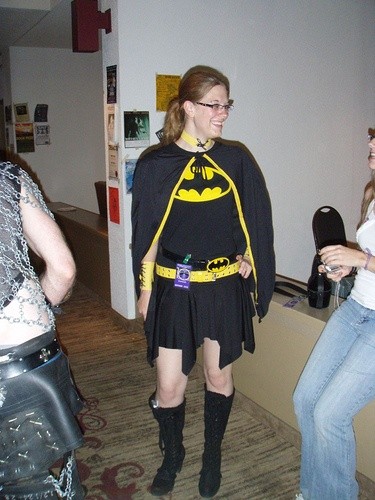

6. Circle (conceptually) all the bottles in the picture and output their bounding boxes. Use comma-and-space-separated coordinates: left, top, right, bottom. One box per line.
308, 254, 333, 309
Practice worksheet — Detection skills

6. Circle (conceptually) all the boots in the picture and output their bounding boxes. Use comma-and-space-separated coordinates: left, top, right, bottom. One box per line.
148, 391, 186, 496
199, 383, 236, 497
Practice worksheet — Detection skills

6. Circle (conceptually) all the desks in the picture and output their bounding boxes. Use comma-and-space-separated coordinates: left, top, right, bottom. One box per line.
46, 202, 111, 303
197, 274, 375, 482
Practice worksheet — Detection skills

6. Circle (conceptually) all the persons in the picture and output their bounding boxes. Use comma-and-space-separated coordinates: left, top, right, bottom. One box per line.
0, 158, 85, 500
128, 65, 276, 497
293, 136, 375, 500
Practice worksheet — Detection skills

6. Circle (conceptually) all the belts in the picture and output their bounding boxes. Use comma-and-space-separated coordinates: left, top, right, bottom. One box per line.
0, 337, 61, 382
155, 257, 240, 283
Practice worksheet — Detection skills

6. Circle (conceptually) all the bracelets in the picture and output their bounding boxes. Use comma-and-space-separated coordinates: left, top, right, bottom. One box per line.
364, 248, 371, 270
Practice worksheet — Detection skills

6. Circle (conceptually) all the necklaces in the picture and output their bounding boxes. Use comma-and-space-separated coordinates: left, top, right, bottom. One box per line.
181, 131, 211, 148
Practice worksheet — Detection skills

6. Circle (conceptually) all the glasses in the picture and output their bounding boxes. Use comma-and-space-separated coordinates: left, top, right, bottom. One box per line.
368, 128, 375, 138
196, 102, 234, 112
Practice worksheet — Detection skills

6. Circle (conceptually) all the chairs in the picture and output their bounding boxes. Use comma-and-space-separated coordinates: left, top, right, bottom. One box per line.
312, 206, 347, 251
